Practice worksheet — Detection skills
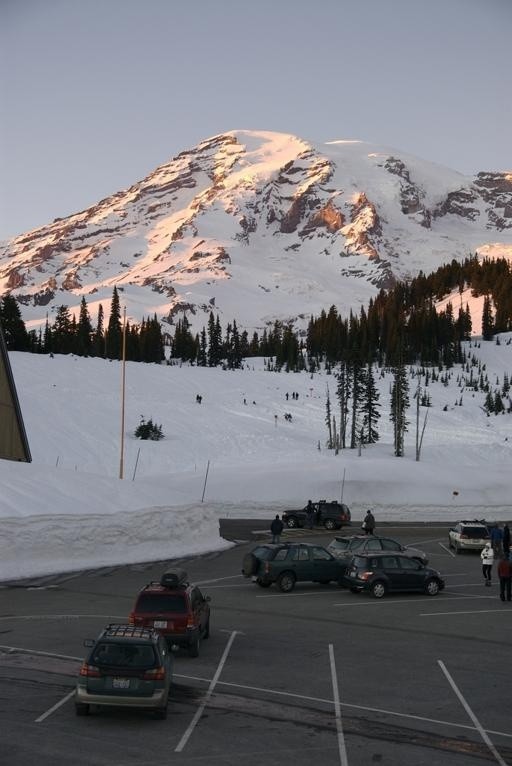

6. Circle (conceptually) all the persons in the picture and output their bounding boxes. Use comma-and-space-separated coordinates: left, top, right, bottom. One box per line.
481, 543, 494, 587
490, 524, 511, 601
364, 509, 376, 535
271, 514, 283, 542
307, 499, 315, 530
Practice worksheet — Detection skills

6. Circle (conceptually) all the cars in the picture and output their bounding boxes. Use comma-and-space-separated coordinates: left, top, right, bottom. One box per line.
448, 521, 492, 554
241, 534, 446, 599
75, 567, 211, 720
282, 500, 351, 529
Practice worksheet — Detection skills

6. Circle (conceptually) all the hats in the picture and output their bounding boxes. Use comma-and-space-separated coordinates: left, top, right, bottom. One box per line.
486, 543, 492, 548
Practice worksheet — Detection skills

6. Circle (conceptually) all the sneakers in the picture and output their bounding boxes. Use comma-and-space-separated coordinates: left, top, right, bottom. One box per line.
484, 578, 492, 587
